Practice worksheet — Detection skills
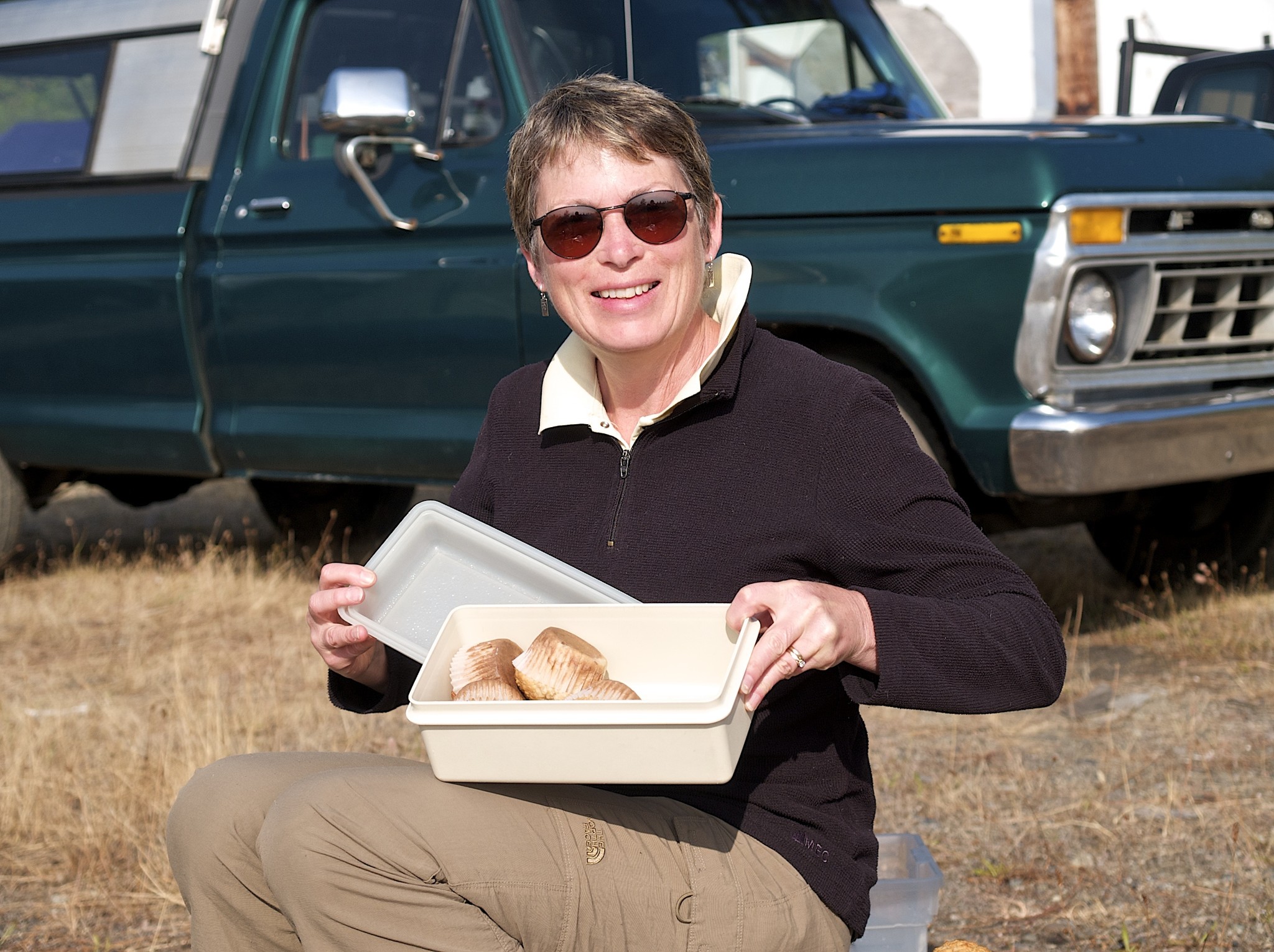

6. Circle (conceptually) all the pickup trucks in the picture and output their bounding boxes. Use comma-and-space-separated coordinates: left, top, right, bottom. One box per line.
1, 3, 1274, 584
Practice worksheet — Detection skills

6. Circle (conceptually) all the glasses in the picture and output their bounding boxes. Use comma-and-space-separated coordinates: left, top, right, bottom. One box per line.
528, 190, 705, 262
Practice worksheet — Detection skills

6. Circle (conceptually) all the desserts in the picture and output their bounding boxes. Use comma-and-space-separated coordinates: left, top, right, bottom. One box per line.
447, 624, 642, 700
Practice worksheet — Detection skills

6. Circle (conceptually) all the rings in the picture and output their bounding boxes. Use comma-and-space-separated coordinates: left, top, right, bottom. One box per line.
788, 644, 808, 671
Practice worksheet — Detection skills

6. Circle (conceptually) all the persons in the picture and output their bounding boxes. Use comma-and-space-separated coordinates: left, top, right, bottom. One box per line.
167, 64, 1072, 952
